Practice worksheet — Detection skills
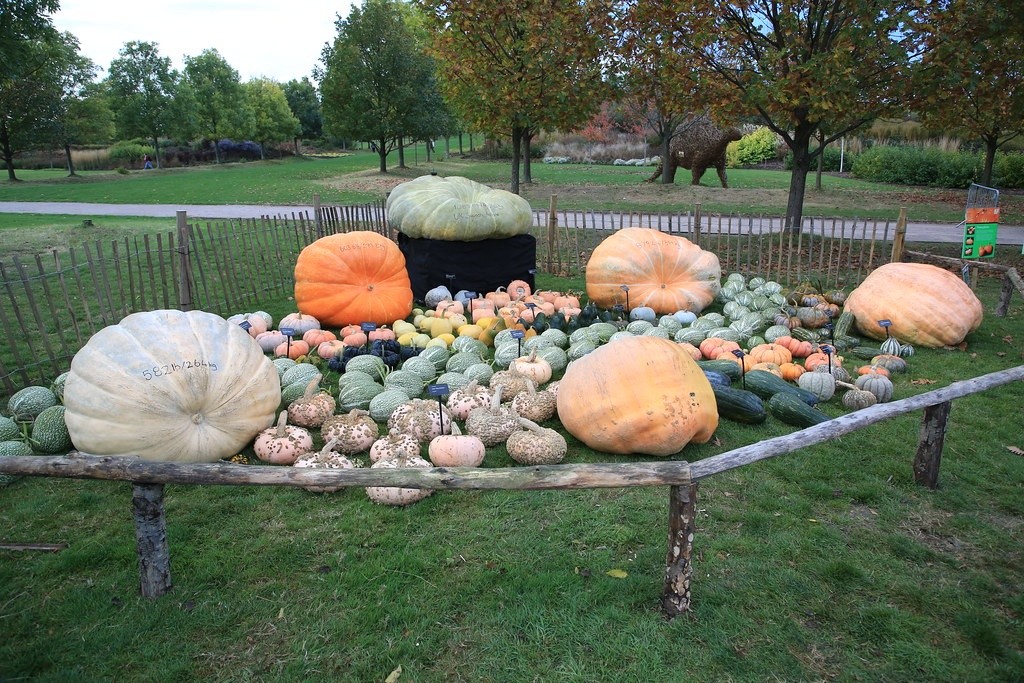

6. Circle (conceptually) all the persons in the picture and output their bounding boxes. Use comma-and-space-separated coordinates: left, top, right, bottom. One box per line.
429, 138, 435, 153
371, 142, 376, 152
144, 153, 153, 169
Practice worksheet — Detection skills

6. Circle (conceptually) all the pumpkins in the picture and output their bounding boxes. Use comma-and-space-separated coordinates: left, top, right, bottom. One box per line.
0, 172, 984, 510
